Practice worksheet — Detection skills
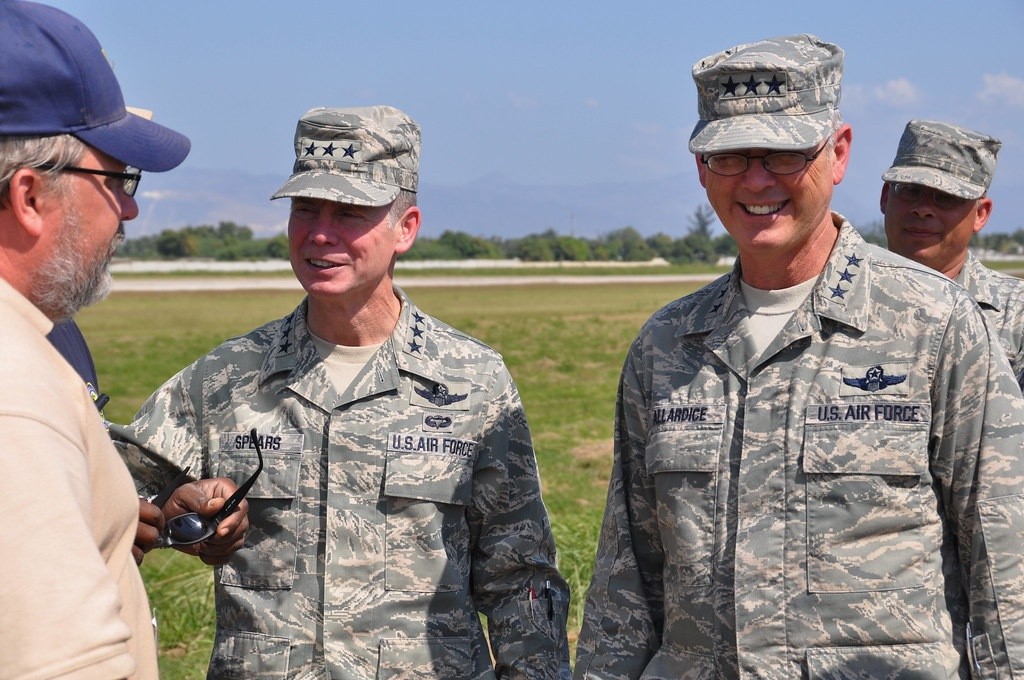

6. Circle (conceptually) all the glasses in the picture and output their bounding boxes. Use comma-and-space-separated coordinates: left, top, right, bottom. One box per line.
150, 427, 263, 549
699, 131, 835, 176
29, 161, 143, 199
889, 181, 975, 207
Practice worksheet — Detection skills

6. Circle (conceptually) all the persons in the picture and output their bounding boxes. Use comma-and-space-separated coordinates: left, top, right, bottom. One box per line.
878, 116, 1023, 417
570, 33, 1024, 680
75, 102, 577, 680
2, 3, 193, 680
39, 305, 252, 567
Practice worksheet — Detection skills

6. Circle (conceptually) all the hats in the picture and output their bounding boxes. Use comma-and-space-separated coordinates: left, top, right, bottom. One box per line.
0, 0, 192, 173
881, 118, 1002, 200
270, 104, 421, 209
689, 33, 846, 152
